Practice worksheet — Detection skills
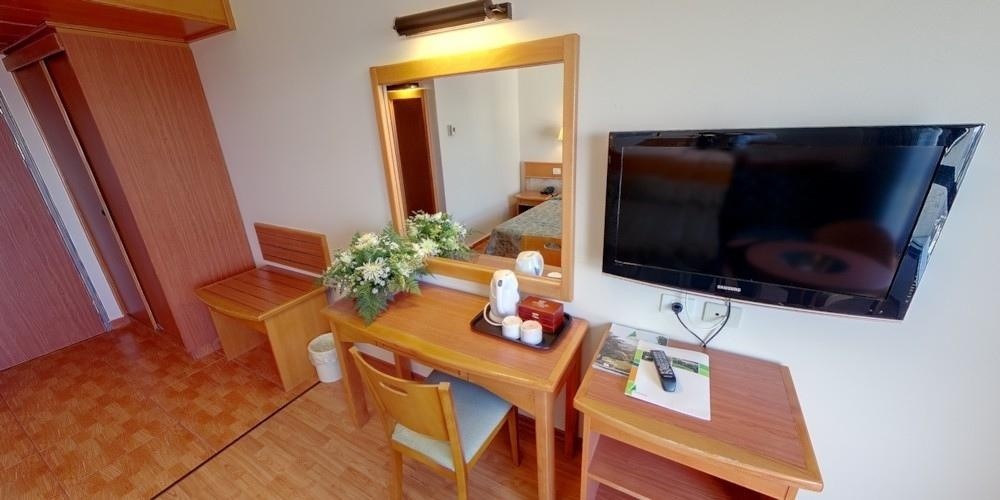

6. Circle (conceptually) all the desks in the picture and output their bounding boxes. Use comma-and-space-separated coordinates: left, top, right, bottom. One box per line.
476, 252, 562, 282
319, 276, 590, 500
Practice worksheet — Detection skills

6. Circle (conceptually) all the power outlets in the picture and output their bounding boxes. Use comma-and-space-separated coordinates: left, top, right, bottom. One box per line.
660, 294, 697, 319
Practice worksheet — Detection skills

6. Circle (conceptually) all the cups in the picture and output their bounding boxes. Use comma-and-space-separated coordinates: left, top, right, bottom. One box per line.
501, 315, 523, 340
548, 272, 561, 279
521, 320, 543, 345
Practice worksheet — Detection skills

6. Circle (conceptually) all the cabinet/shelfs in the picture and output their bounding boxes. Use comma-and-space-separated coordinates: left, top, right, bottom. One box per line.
194, 263, 333, 391
573, 319, 823, 500
0, 26, 256, 362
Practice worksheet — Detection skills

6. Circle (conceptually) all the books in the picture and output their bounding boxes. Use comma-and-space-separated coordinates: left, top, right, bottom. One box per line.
625, 339, 711, 421
590, 323, 670, 379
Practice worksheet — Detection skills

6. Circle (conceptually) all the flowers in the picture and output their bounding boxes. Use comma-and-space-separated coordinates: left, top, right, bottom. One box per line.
320, 229, 441, 327
404, 208, 486, 264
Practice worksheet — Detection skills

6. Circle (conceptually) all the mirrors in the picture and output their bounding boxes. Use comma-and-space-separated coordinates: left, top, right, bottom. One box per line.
369, 33, 579, 303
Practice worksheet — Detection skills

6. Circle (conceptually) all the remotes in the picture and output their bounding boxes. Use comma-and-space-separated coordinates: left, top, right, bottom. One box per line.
651, 350, 677, 393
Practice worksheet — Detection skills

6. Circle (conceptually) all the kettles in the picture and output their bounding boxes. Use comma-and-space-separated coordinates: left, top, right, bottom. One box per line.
488, 270, 520, 323
516, 250, 544, 277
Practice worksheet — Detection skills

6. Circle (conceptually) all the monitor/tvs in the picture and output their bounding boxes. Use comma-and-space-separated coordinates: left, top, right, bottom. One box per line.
601, 123, 989, 320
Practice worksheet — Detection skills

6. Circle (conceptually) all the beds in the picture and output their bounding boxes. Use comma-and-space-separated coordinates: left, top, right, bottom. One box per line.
485, 192, 562, 259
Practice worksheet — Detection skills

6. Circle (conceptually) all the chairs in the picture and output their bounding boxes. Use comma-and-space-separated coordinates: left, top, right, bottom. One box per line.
348, 345, 520, 500
519, 234, 562, 267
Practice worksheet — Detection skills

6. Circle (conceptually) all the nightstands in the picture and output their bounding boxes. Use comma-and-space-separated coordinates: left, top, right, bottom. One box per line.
514, 188, 560, 216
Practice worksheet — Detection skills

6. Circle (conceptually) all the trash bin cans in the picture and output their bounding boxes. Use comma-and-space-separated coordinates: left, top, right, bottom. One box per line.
307, 332, 343, 383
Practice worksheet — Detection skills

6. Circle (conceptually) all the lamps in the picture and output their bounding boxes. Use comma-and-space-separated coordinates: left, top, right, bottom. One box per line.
392, 0, 513, 41
558, 127, 563, 141
